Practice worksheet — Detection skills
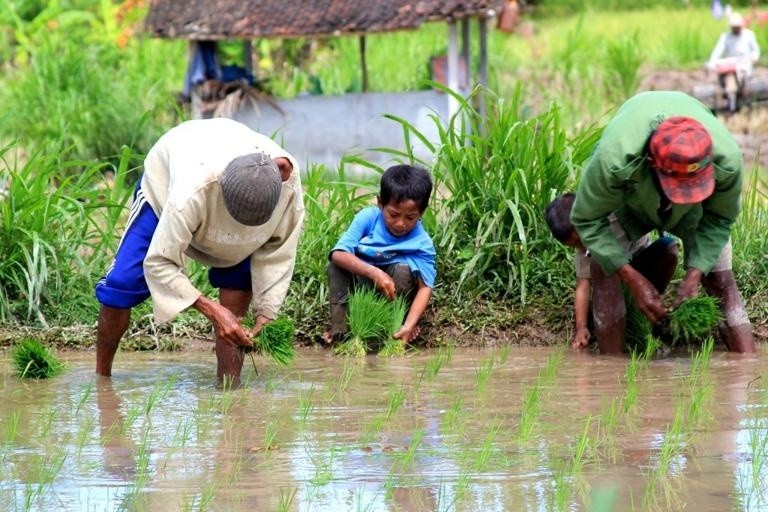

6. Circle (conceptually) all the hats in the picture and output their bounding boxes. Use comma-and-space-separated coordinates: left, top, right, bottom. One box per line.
650, 116, 715, 206
220, 152, 282, 226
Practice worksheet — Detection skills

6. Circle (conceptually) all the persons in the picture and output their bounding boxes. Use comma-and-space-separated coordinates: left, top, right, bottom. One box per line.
497, 0, 520, 34
171, 38, 233, 106
567, 87, 758, 359
574, 365, 745, 512
331, 363, 442, 512
94, 115, 305, 392
706, 11, 760, 115
96, 396, 284, 512
323, 162, 438, 352
541, 191, 678, 351
